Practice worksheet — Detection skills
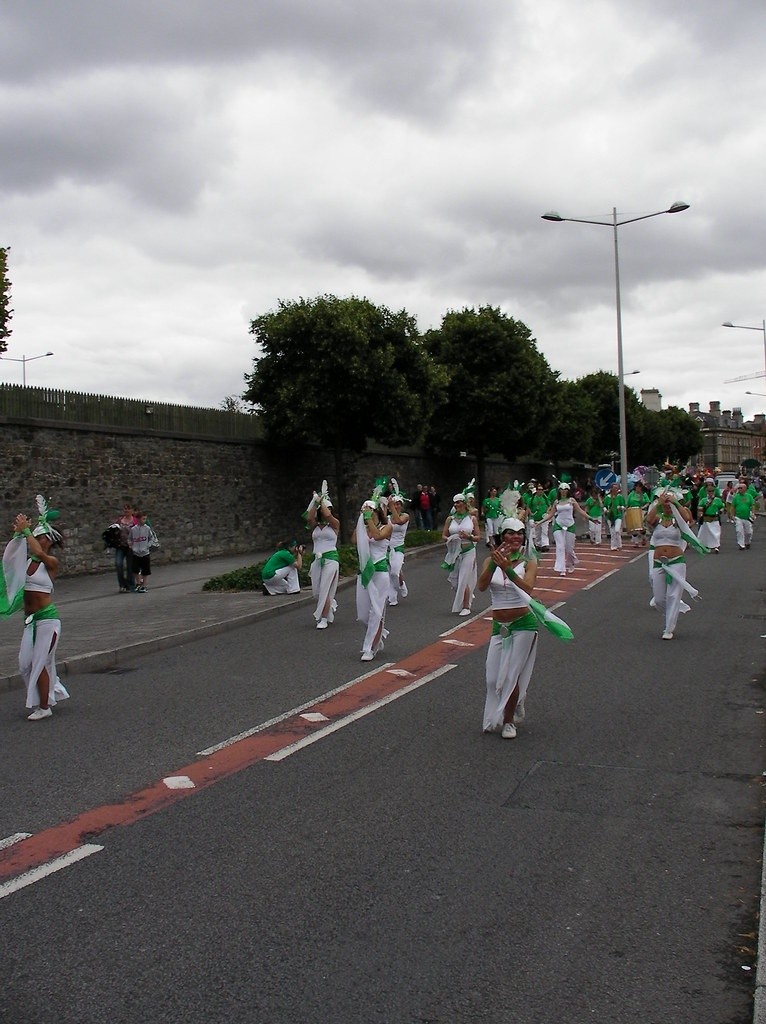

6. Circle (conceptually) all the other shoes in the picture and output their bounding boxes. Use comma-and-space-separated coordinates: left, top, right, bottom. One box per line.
650, 596, 657, 607
568, 564, 575, 573
501, 723, 517, 738
288, 591, 301, 595
115, 581, 150, 593
484, 517, 754, 555
360, 649, 378, 661
327, 613, 335, 624
316, 622, 328, 629
399, 580, 409, 598
262, 583, 276, 596
560, 572, 566, 576
662, 632, 674, 640
27, 706, 53, 720
459, 609, 472, 616
389, 601, 398, 606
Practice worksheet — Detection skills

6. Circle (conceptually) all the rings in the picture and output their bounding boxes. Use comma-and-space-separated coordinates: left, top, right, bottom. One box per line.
501, 548, 505, 552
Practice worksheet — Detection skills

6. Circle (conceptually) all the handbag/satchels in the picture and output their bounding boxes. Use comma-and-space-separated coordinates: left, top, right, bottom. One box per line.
698, 516, 704, 525
725, 489, 736, 503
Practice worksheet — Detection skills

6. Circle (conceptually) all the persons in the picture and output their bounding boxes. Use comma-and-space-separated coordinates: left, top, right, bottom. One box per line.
730, 484, 755, 550
261, 538, 303, 596
645, 474, 711, 640
533, 472, 602, 577
301, 480, 340, 629
115, 503, 154, 593
696, 486, 725, 554
472, 479, 574, 739
440, 478, 480, 616
351, 470, 766, 662
351, 475, 393, 662
0, 494, 70, 721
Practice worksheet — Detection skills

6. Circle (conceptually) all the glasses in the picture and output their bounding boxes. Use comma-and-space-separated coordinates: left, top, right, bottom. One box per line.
451, 500, 463, 504
706, 489, 715, 493
538, 489, 544, 491
736, 487, 743, 490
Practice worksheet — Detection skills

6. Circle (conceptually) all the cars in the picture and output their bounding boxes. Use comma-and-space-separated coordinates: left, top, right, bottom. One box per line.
705, 471, 740, 498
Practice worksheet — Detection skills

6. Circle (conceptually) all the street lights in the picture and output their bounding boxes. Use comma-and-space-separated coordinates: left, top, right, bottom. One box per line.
541, 201, 690, 529
722, 319, 766, 376
0, 352, 54, 385
